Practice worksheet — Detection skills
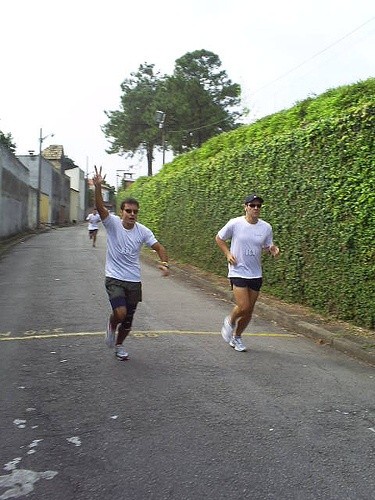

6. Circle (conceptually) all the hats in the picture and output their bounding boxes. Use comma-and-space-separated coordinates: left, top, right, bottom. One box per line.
245, 195, 264, 205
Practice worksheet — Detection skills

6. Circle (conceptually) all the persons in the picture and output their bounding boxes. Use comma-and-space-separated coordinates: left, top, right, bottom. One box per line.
86, 209, 101, 248
215, 195, 279, 352
92, 165, 170, 360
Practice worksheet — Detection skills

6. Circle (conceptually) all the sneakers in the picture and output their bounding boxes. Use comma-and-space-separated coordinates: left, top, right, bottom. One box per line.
112, 343, 129, 360
104, 318, 116, 348
230, 335, 247, 352
221, 315, 235, 343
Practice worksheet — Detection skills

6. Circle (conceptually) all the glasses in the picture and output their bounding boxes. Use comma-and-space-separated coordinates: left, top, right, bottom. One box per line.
124, 209, 138, 214
248, 204, 262, 208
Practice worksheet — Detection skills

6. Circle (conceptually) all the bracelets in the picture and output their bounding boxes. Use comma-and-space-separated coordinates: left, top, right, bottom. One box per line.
161, 261, 168, 266
